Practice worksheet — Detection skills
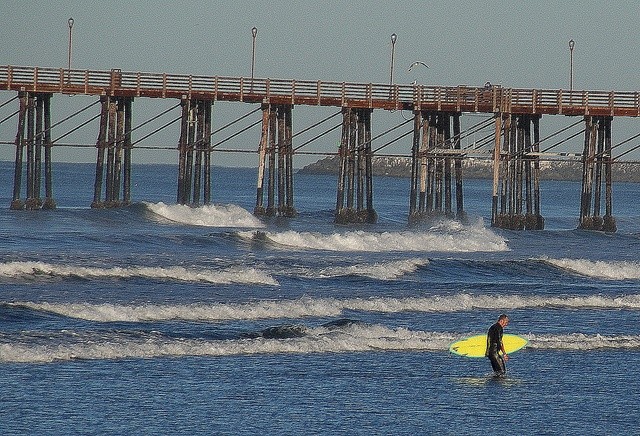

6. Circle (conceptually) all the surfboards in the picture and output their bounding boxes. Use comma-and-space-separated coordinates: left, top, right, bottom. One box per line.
449, 333, 528, 358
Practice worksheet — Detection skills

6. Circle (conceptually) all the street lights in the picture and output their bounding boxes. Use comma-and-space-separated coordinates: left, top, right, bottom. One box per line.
67, 16, 76, 85
249, 25, 258, 96
569, 40, 576, 106
388, 33, 398, 101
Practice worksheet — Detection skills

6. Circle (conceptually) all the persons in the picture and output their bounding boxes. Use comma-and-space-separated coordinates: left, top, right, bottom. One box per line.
485, 314, 510, 377
